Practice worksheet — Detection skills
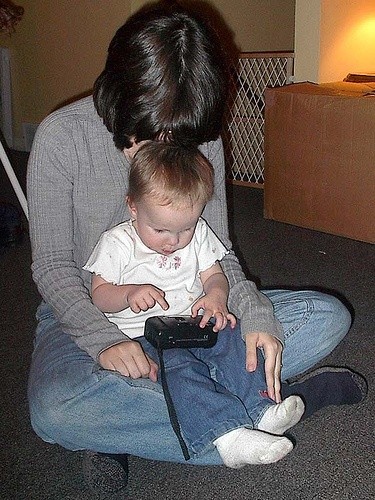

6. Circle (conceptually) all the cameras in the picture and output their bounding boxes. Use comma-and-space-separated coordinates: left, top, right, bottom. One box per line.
144, 315, 218, 350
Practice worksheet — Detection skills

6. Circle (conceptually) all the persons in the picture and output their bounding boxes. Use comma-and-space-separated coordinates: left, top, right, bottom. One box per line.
80, 142, 305, 469
25, 6, 367, 491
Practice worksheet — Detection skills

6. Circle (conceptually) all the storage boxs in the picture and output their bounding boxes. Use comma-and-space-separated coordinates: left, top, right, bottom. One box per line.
263, 73, 375, 245
